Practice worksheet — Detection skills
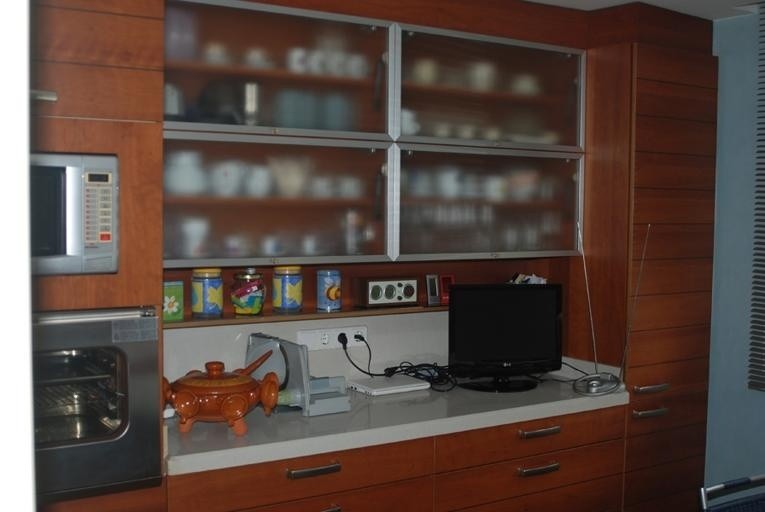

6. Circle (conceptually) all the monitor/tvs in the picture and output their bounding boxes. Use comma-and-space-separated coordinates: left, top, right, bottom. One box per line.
448, 283, 563, 394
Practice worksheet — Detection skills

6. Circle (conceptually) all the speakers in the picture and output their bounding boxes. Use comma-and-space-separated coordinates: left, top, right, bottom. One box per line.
356, 277, 419, 307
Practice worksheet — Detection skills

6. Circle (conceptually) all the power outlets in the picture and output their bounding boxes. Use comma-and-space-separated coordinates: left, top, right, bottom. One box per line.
296, 325, 369, 349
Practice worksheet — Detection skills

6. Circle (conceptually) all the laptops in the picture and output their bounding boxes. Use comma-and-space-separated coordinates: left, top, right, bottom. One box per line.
346, 374, 431, 397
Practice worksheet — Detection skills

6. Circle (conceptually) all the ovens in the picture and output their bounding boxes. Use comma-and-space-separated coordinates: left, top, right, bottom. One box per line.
31, 305, 158, 502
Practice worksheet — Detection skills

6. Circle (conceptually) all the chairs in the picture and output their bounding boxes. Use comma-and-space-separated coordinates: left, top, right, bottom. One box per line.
697, 470, 764, 510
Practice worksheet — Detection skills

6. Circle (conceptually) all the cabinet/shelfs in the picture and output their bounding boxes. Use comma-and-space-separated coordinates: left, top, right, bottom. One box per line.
165, 0, 391, 263
394, 15, 587, 259
167, 434, 439, 512
29, 1, 170, 124
439, 409, 630, 510
564, 4, 711, 511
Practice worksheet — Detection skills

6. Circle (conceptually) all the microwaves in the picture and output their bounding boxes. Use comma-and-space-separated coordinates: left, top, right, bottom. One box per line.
31, 153, 122, 278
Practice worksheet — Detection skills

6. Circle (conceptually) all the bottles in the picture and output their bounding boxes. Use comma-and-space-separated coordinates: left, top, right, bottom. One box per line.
229, 267, 267, 317
189, 266, 222, 320
272, 265, 304, 314
314, 269, 343, 313
